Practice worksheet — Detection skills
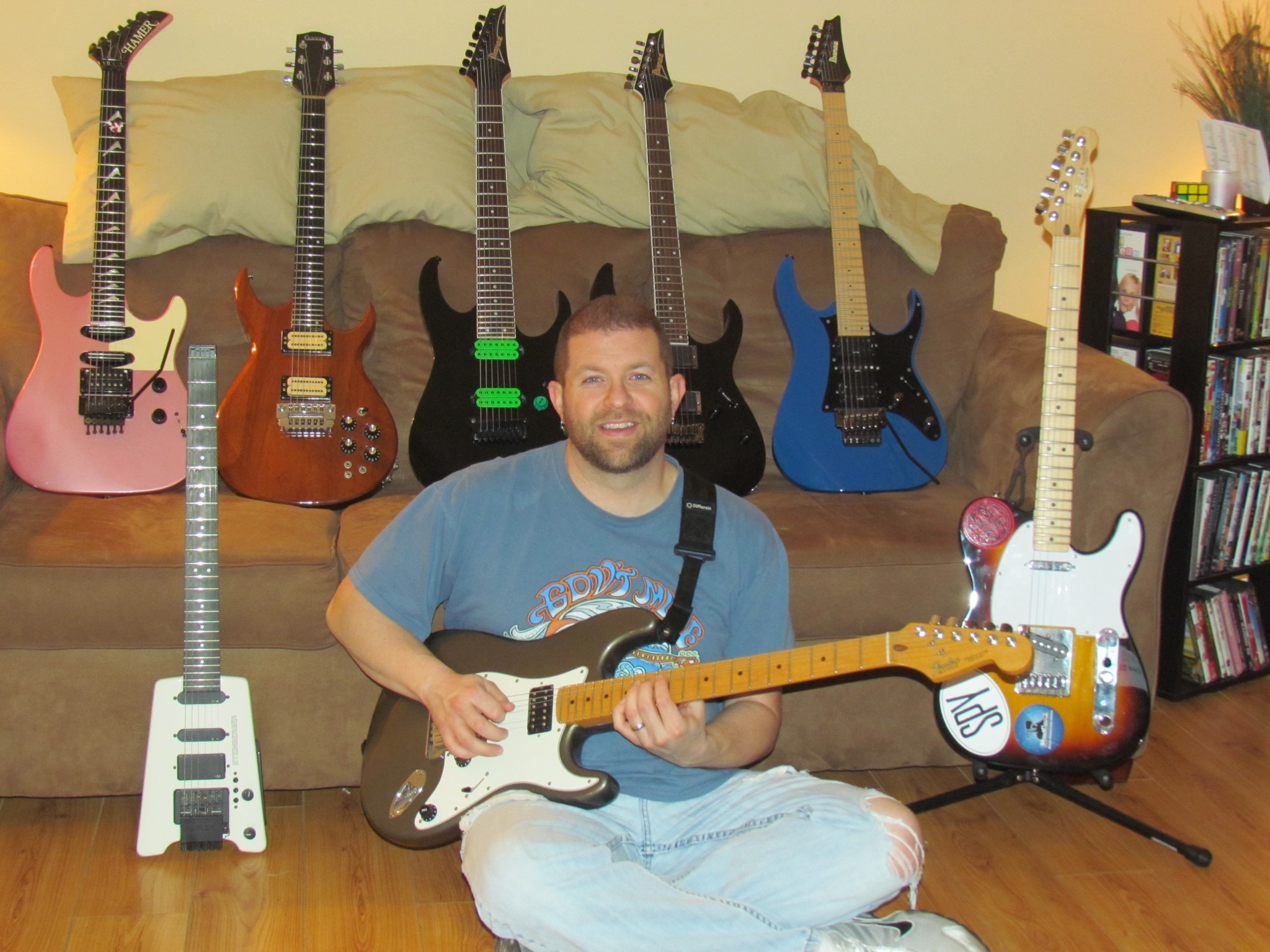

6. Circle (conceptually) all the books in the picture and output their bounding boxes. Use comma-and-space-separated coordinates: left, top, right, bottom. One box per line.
1111, 346, 1270, 463
1111, 227, 1270, 346
1181, 573, 1270, 686
1189, 463, 1270, 582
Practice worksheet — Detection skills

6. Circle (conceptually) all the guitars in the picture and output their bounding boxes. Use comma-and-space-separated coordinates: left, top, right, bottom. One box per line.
407, 4, 571, 488
358, 606, 1034, 852
5, 10, 187, 495
134, 343, 266, 859
935, 126, 1153, 776
216, 32, 398, 505
589, 28, 767, 498
771, 15, 948, 492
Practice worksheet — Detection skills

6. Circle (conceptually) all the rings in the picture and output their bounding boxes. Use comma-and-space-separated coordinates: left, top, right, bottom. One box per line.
631, 723, 646, 731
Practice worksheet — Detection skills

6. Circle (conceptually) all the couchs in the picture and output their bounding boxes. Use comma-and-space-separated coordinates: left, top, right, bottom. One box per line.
0, 185, 1195, 808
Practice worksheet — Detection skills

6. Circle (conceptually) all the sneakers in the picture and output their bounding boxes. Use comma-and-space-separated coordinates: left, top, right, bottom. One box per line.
828, 910, 987, 952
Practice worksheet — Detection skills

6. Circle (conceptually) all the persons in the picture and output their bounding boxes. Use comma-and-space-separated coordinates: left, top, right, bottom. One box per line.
325, 295, 993, 952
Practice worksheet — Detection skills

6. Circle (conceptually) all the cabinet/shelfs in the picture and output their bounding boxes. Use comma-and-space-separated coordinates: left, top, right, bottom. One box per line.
1075, 205, 1270, 706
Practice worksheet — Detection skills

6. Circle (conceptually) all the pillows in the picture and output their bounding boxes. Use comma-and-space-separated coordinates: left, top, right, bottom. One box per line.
48, 64, 514, 268
496, 72, 953, 281
